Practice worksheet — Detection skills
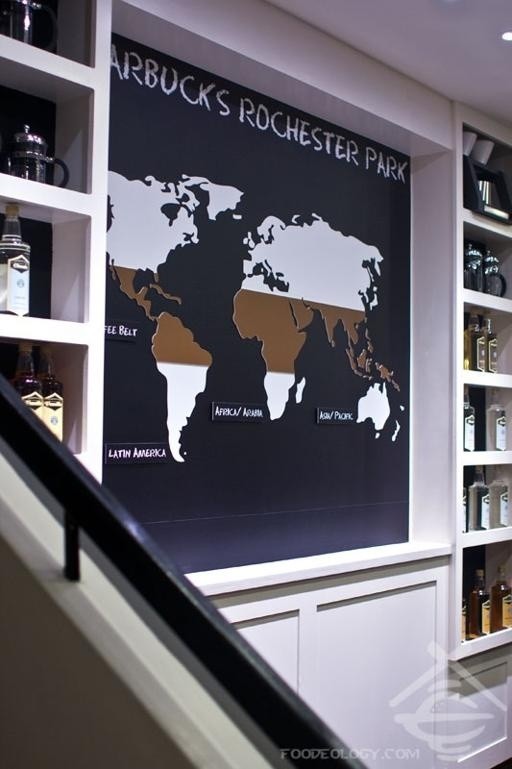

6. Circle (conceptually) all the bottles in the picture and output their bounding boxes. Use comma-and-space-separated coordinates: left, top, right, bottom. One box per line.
461, 309, 512, 642
11, 342, 44, 423
36, 344, 64, 444
0, 203, 31, 318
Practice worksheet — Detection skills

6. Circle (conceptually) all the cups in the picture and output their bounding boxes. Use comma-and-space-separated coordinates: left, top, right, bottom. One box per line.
464, 243, 484, 292
482, 250, 506, 297
6, 124, 70, 187
462, 129, 496, 167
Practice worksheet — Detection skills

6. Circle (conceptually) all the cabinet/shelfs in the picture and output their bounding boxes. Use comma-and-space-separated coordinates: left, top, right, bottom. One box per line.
411, 97, 512, 664
0, 1, 110, 505
